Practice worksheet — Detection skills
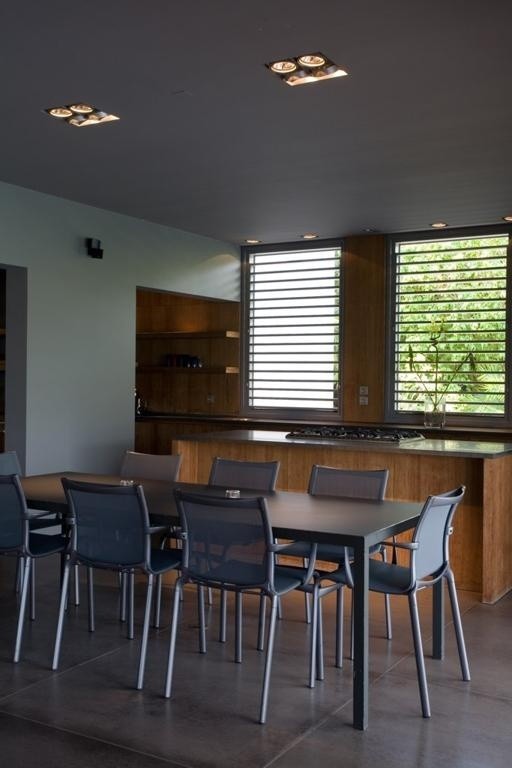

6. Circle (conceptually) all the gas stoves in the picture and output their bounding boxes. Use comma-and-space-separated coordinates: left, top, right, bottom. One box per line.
286, 425, 426, 444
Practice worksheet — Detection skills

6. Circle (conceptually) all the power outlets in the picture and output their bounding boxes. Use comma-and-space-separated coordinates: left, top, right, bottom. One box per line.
360, 385, 368, 396
359, 396, 368, 406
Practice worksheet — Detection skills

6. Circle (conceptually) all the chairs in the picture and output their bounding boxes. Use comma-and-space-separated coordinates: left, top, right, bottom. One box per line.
1, 475, 96, 662
179, 457, 283, 602
161, 492, 319, 723
310, 486, 473, 719
52, 476, 207, 689
119, 451, 183, 482
273, 464, 390, 682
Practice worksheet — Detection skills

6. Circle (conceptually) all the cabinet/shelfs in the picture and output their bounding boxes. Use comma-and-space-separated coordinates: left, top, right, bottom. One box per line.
135, 286, 241, 456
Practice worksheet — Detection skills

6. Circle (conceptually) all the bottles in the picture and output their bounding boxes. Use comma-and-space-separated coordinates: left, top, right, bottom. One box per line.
164, 352, 205, 370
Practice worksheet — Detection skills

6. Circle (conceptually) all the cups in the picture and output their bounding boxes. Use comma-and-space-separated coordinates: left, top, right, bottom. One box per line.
225, 490, 240, 498
120, 479, 135, 487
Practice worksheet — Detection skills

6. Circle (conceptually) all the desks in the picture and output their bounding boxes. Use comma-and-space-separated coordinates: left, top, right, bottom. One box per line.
2, 470, 429, 730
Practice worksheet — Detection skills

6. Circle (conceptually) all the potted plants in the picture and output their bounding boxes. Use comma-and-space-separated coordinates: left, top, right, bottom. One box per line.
401, 317, 488, 429
